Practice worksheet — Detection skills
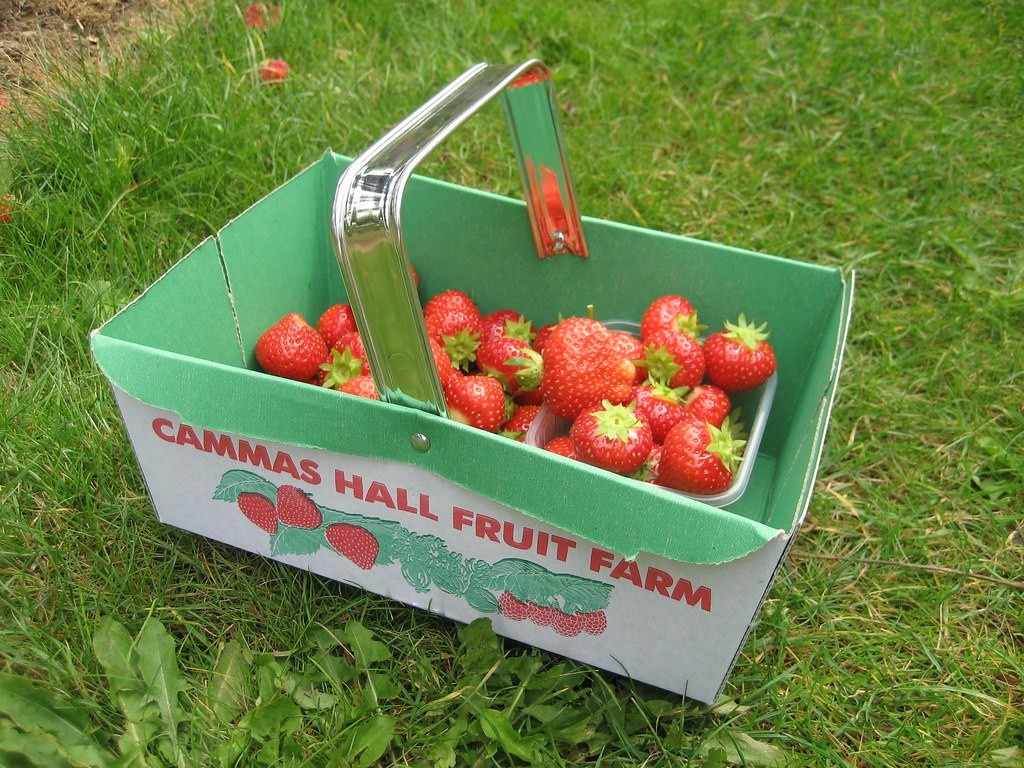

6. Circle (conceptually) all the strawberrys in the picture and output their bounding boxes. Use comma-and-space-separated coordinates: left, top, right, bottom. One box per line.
255, 262, 776, 497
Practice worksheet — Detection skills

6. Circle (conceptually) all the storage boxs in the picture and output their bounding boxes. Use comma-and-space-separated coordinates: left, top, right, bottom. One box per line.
88, 57, 854, 708
